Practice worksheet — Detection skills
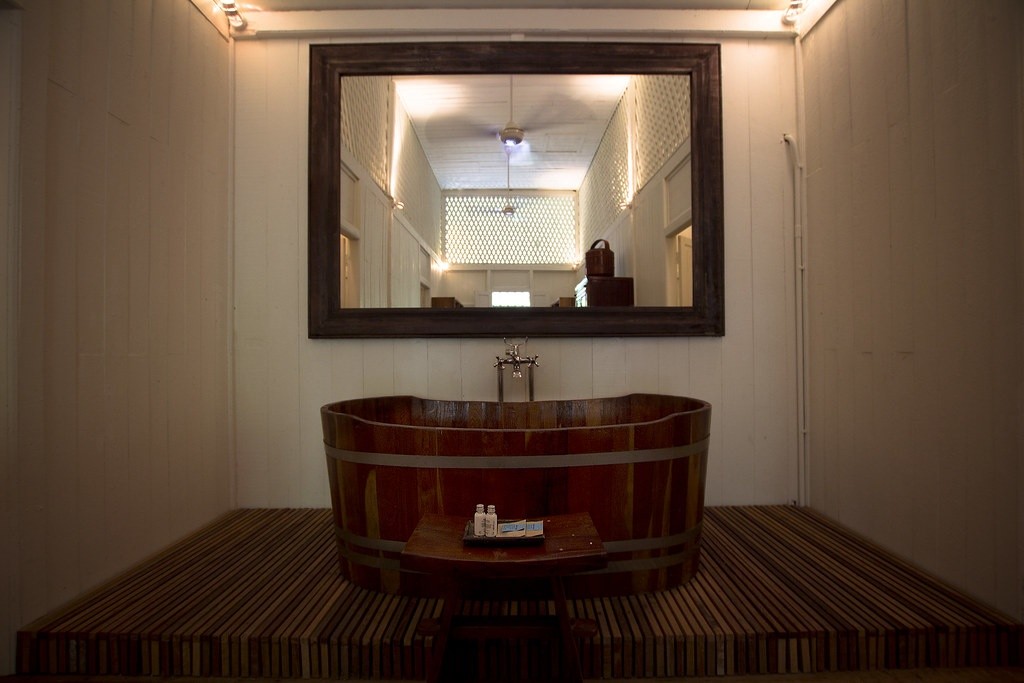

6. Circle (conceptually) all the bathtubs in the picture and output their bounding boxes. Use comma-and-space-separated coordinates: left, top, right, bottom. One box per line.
318, 388, 714, 602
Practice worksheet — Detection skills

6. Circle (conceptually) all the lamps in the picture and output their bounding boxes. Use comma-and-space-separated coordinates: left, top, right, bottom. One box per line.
392, 199, 405, 210
500, 117, 525, 145
503, 205, 515, 215
618, 201, 631, 212
781, 0, 804, 24
222, 0, 249, 32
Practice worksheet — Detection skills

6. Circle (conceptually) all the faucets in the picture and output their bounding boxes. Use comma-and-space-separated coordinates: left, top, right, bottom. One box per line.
504, 339, 526, 383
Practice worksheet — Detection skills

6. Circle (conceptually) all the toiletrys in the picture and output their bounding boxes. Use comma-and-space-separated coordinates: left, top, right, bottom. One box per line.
484, 505, 498, 538
474, 503, 487, 537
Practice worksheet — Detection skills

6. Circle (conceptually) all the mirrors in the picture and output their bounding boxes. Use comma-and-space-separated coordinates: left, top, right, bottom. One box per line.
307, 40, 726, 340
